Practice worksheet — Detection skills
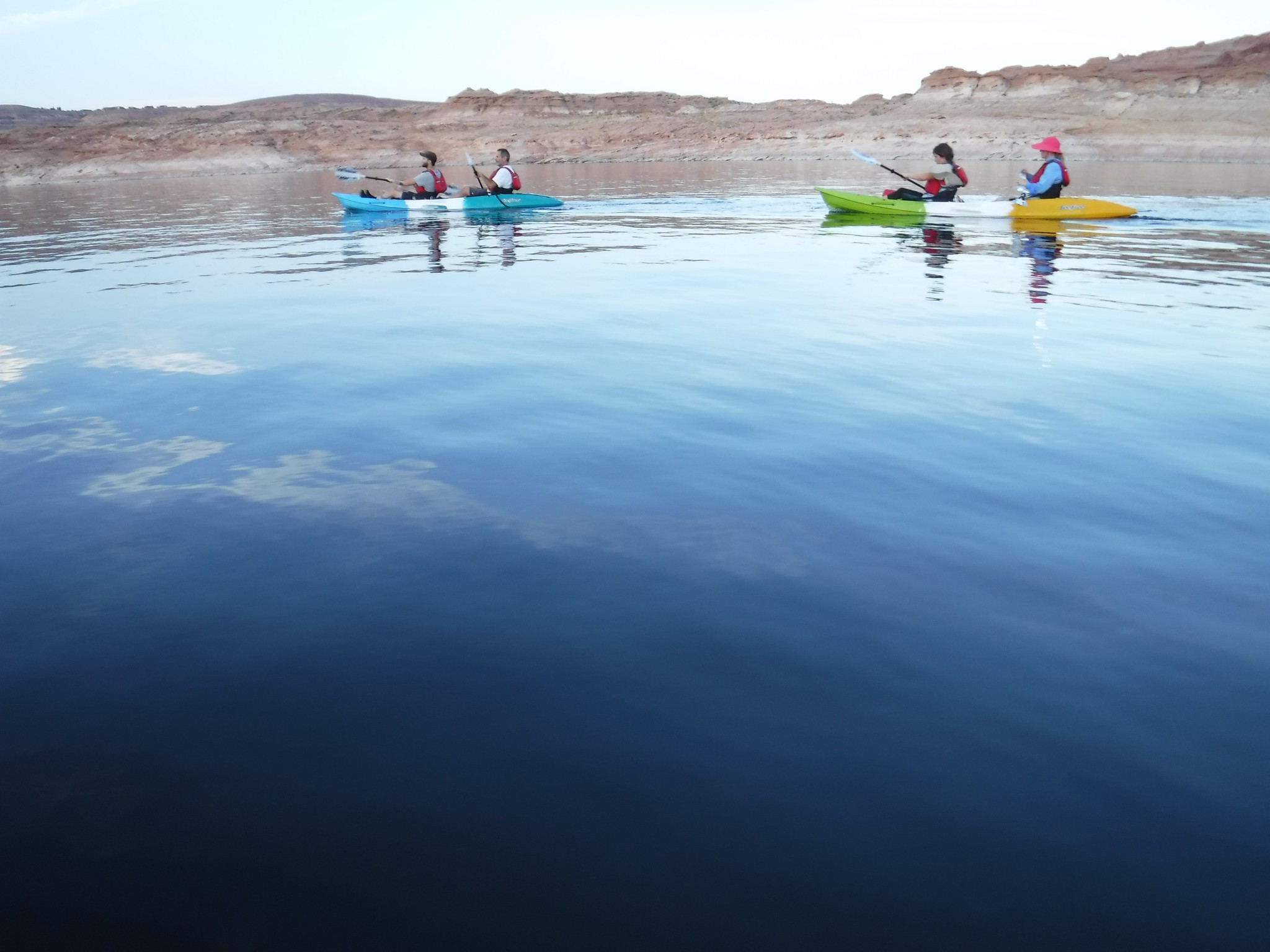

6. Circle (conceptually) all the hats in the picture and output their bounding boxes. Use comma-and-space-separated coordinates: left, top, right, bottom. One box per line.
419, 151, 437, 163
1031, 137, 1063, 154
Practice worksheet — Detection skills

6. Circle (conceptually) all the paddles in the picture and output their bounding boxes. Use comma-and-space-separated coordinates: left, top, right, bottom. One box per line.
848, 147, 927, 190
1013, 173, 1030, 207
465, 149, 485, 190
335, 165, 460, 196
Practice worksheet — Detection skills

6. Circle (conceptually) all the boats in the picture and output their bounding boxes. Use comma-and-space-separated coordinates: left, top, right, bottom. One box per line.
331, 192, 567, 212
812, 186, 1138, 221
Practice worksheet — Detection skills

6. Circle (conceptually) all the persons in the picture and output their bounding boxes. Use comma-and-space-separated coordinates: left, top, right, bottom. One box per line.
360, 151, 443, 200
994, 137, 1065, 202
430, 149, 515, 199
880, 143, 957, 202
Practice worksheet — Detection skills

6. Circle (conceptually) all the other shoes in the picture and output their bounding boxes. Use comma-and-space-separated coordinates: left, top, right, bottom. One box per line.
360, 189, 369, 198
366, 190, 377, 198
